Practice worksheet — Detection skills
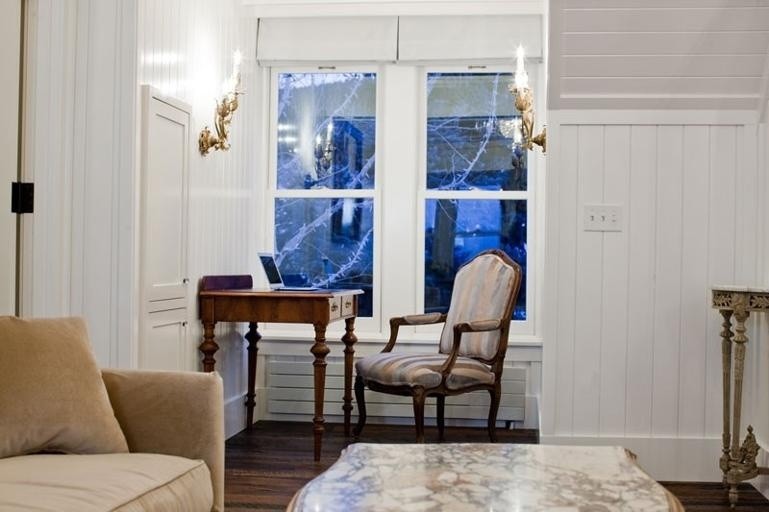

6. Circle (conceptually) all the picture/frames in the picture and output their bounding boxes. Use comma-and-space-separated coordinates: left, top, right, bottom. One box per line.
331, 117, 365, 243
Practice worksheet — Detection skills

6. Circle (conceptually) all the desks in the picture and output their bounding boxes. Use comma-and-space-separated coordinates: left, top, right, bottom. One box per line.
200, 289, 363, 469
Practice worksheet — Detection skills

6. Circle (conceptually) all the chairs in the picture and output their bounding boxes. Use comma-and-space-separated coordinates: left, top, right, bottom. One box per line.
353, 249, 522, 443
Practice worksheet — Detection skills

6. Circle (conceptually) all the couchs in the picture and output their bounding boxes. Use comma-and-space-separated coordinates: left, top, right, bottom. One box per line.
1, 314, 225, 510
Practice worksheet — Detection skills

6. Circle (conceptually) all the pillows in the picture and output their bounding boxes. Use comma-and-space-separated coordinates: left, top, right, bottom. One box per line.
0, 315, 129, 459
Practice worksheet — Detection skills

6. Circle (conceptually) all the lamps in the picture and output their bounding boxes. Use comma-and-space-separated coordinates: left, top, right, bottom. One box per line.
508, 44, 548, 154
199, 50, 245, 158
304, 121, 350, 190
511, 124, 527, 192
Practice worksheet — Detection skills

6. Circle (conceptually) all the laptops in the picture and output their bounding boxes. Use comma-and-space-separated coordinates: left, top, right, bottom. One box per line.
258, 251, 321, 291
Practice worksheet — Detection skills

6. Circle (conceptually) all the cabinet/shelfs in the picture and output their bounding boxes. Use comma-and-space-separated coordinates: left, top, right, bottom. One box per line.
711, 285, 769, 508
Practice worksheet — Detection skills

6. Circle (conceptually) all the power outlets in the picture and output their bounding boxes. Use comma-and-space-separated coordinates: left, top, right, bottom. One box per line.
582, 205, 623, 231
